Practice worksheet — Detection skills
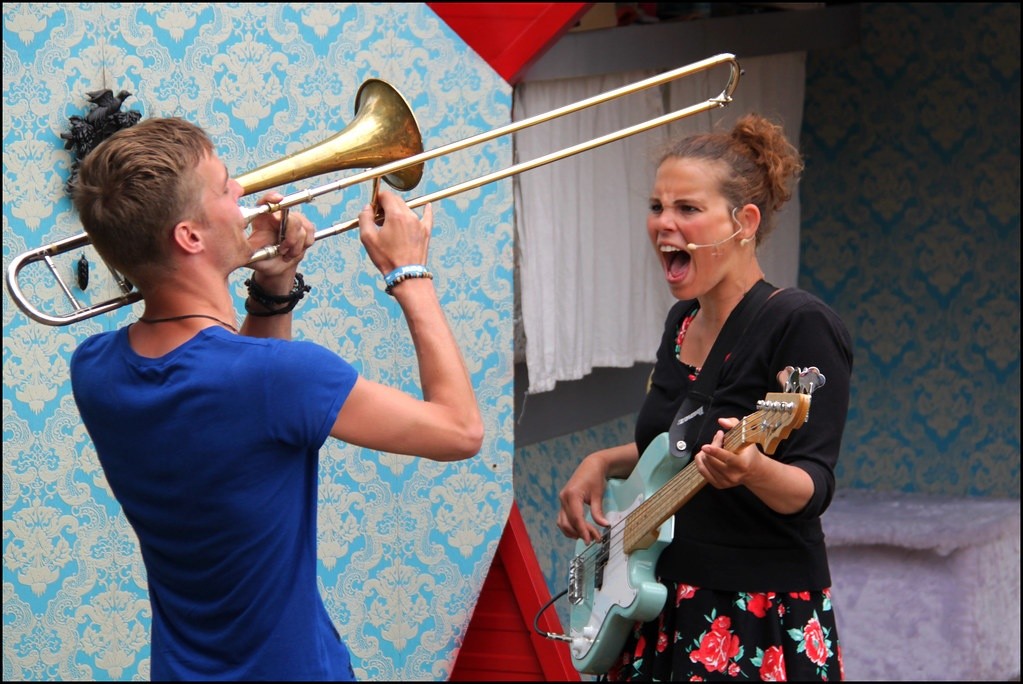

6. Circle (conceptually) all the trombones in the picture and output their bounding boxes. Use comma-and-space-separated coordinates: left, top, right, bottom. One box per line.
3, 47, 743, 332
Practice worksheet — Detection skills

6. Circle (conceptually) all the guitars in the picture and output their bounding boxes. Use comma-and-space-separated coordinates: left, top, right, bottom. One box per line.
564, 364, 829, 677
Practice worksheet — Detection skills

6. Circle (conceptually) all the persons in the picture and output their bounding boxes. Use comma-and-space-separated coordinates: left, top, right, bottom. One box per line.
67, 117, 485, 682
558, 113, 854, 683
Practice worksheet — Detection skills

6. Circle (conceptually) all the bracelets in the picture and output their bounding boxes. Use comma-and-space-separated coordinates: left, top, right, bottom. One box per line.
384, 264, 433, 295
243, 270, 312, 316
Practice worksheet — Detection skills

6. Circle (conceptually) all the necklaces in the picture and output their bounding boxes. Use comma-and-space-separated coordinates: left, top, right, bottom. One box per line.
139, 314, 237, 331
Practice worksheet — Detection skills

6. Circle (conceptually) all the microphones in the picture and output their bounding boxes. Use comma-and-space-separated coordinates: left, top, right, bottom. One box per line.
686, 207, 756, 250
